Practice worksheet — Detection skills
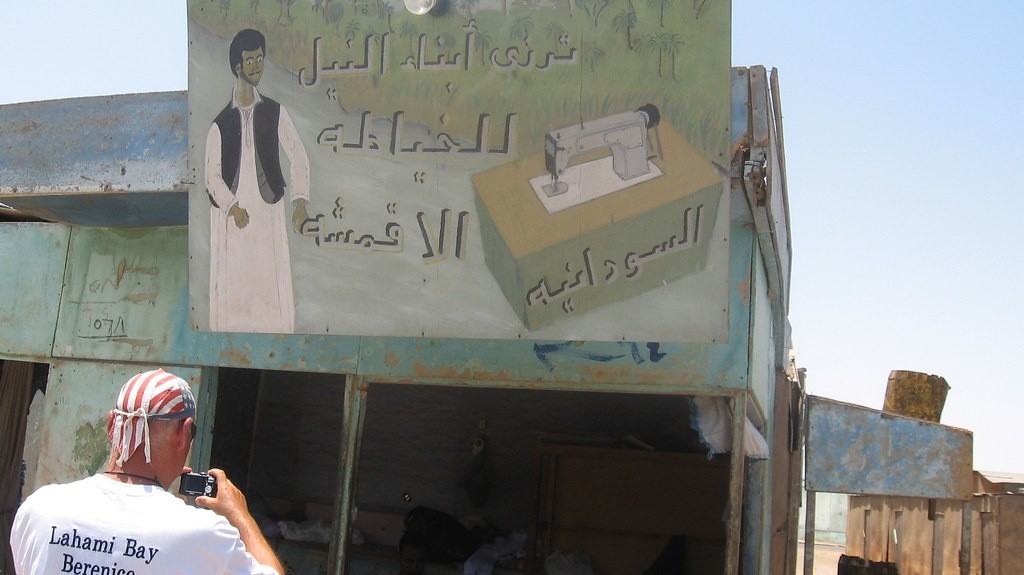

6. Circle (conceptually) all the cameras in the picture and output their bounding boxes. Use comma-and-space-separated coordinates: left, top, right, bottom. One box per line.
178, 470, 219, 498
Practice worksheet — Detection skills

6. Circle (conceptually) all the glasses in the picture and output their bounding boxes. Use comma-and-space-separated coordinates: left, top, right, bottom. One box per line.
179, 419, 197, 441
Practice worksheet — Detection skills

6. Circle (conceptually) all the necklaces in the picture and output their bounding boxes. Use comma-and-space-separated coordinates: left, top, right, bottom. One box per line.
103, 471, 161, 486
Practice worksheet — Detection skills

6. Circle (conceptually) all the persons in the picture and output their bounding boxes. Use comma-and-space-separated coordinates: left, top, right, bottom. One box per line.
379, 533, 428, 575
9, 368, 285, 575
